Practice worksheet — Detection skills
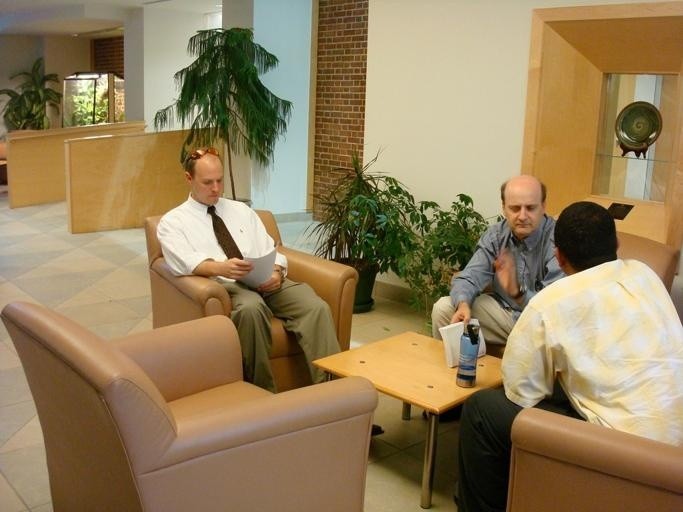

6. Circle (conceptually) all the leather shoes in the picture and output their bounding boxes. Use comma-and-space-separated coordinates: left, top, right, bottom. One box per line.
370, 423, 384, 440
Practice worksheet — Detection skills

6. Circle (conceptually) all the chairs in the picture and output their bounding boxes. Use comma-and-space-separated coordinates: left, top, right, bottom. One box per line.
142, 205, 359, 393
502, 405, 683, 512
615, 231, 680, 296
1, 300, 378, 512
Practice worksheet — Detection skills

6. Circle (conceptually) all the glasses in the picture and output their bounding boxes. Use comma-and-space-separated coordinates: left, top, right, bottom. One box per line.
186, 148, 218, 160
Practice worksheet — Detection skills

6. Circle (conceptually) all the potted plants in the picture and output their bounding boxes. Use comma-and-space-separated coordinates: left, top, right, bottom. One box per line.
346, 178, 489, 317
288, 144, 409, 316
154, 25, 294, 209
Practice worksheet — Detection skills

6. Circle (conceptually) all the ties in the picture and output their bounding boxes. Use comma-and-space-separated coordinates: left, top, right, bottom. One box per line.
207, 205, 243, 261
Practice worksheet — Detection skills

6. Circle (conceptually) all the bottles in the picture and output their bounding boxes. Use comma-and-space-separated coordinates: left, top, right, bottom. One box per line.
456, 324, 480, 390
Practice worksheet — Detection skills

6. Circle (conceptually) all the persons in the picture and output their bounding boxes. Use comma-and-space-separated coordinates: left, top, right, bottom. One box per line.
156, 148, 385, 437
421, 172, 571, 423
455, 198, 683, 512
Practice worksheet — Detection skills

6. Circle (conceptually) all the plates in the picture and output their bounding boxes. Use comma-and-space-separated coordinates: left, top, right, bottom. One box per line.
615, 101, 663, 150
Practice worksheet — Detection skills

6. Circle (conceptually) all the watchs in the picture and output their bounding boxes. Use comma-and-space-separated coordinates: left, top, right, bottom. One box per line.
511, 282, 529, 300
275, 268, 286, 283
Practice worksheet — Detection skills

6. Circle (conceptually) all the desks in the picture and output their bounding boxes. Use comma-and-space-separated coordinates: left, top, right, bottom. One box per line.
309, 330, 517, 512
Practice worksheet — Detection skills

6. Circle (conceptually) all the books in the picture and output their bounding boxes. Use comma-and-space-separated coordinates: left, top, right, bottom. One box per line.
438, 317, 488, 369
